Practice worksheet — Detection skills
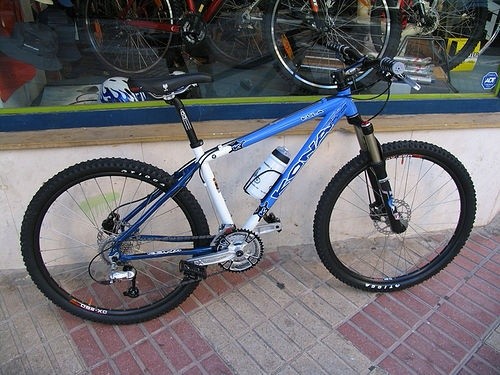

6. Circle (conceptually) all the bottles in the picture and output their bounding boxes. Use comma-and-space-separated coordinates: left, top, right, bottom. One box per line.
245, 146, 291, 199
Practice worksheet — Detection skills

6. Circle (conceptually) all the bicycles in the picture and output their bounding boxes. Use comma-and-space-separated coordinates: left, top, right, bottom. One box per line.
60, 0, 499, 93
19, 39, 478, 324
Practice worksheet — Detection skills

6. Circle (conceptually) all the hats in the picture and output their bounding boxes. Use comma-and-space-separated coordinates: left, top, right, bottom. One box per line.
35, 6, 82, 61
0, 21, 63, 71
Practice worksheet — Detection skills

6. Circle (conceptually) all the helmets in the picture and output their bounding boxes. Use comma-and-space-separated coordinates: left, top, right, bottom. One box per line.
98, 76, 150, 102
